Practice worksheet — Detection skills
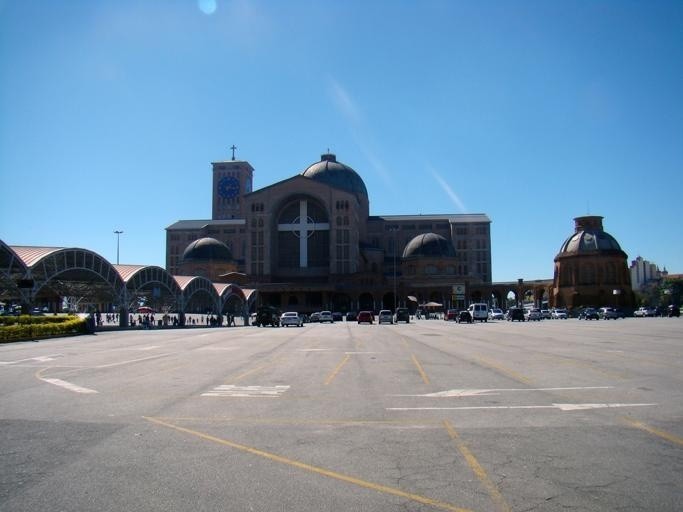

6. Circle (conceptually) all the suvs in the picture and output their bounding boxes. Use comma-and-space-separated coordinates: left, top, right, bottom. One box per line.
255, 305, 280, 328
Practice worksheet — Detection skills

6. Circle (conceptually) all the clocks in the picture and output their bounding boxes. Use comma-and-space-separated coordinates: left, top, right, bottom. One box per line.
216, 175, 240, 200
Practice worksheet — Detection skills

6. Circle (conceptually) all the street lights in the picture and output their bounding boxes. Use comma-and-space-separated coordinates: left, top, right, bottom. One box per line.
662, 289, 667, 307
612, 288, 623, 305
113, 230, 124, 264
385, 223, 401, 309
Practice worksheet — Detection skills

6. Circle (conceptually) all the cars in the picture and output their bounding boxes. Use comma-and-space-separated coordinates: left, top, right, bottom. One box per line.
136, 307, 153, 312
308, 309, 395, 325
445, 302, 681, 324
0, 302, 50, 316
279, 311, 305, 328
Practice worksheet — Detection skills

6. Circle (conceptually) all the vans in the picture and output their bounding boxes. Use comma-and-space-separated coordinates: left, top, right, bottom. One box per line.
394, 308, 410, 323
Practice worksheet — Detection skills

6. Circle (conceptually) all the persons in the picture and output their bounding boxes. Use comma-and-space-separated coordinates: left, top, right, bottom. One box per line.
95, 309, 279, 328
